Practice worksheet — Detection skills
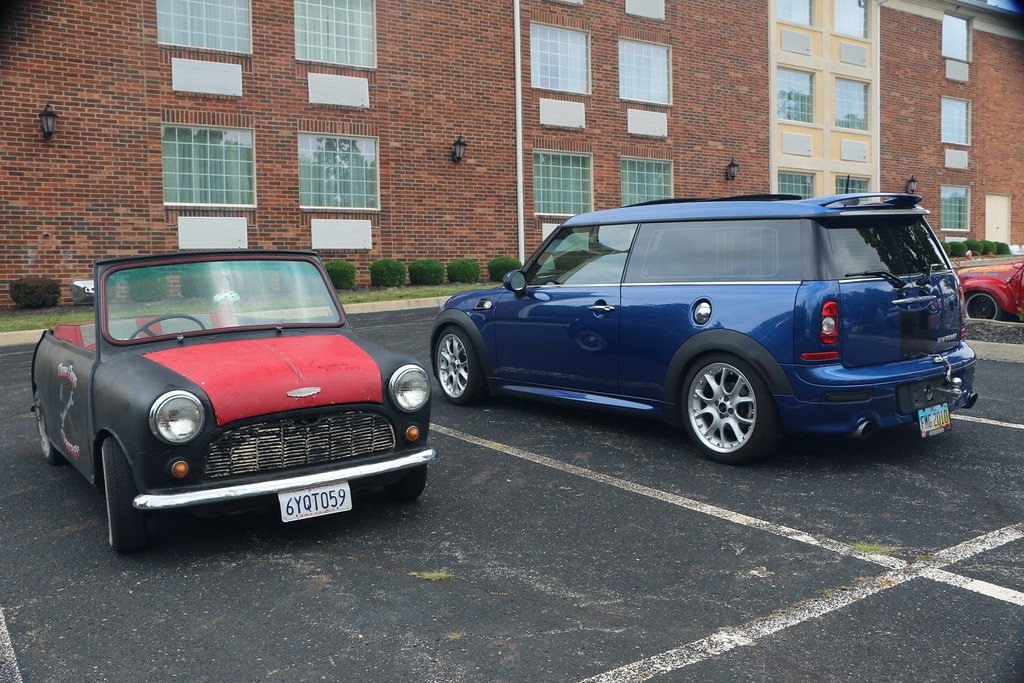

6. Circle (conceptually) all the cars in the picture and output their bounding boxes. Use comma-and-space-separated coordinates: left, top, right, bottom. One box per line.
31, 248, 436, 553
955, 259, 1024, 324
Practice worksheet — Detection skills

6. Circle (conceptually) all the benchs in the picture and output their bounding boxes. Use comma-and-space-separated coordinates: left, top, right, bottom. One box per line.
53, 311, 239, 351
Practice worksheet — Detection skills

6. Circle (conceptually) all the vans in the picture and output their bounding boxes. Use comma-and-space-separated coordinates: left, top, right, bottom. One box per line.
431, 191, 979, 464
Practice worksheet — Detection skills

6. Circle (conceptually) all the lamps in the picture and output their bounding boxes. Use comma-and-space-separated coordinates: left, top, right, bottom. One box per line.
38, 104, 59, 140
905, 174, 918, 194
725, 156, 739, 181
451, 134, 468, 164
